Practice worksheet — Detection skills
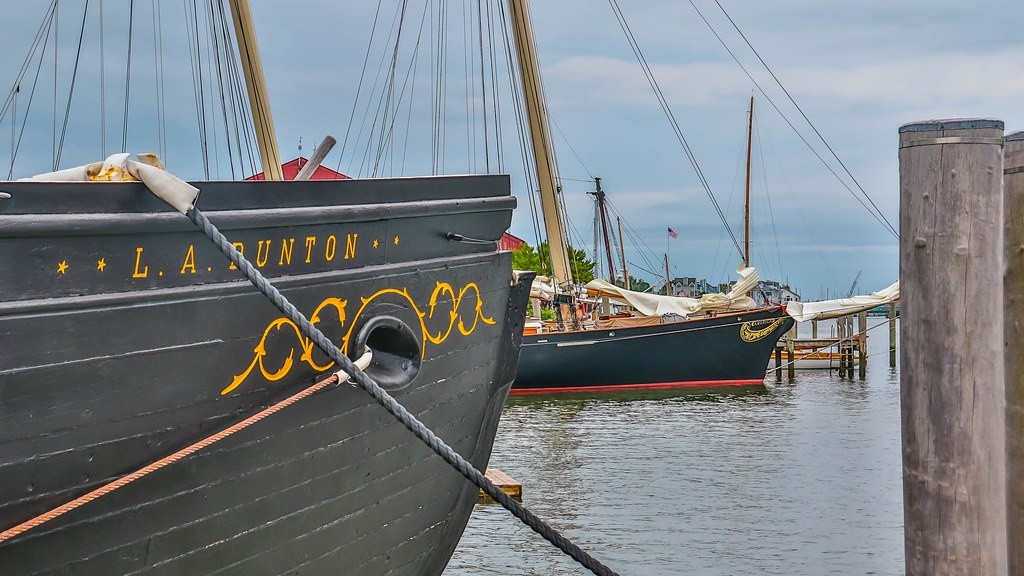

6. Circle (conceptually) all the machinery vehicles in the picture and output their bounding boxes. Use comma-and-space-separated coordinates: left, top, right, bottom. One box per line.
845, 271, 863, 298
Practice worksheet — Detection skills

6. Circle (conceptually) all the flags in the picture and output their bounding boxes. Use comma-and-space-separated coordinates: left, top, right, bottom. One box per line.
668, 227, 678, 239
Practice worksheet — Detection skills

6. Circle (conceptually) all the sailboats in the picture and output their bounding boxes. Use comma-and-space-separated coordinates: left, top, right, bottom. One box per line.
0, 0, 541, 576
335, 0, 866, 407
